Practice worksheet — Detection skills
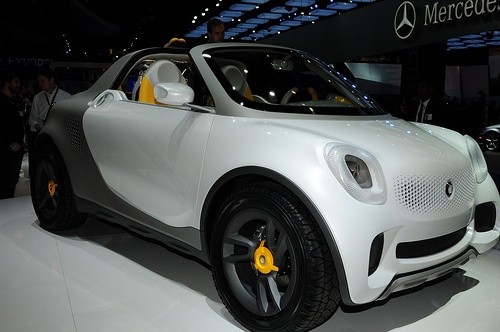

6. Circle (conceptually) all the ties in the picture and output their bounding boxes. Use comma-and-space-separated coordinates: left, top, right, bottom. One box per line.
418, 102, 424, 122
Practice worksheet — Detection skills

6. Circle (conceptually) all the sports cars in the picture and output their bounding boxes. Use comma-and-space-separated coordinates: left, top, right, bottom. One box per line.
27, 43, 500, 332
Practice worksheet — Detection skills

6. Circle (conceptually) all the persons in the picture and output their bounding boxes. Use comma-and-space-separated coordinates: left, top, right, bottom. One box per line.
0, 75, 36, 200
207, 19, 224, 43
406, 77, 440, 125
29, 68, 72, 132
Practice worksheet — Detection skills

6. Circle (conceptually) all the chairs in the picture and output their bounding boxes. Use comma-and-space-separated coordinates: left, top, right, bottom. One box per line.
137, 59, 190, 106
207, 65, 255, 107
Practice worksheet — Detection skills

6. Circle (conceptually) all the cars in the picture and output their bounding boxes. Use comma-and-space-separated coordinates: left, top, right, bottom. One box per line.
477, 123, 500, 153
